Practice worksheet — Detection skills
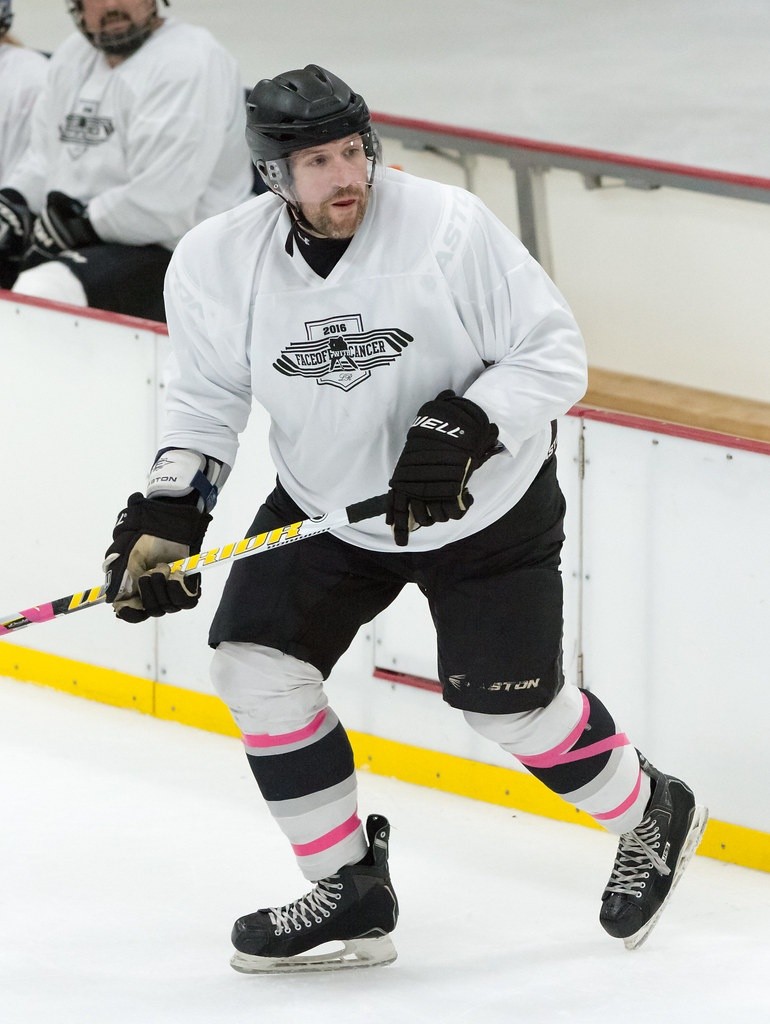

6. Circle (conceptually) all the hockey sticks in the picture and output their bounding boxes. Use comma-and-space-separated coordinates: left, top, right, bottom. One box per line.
0, 489, 395, 641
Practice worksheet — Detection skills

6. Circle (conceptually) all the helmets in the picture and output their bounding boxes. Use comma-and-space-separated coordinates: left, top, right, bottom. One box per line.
242, 62, 384, 169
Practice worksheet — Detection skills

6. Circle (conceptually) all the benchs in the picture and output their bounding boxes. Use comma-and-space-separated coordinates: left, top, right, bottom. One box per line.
577, 368, 770, 449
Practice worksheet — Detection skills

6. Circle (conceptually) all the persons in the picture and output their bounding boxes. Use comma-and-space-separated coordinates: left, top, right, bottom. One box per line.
103, 63, 710, 975
0, 0, 253, 323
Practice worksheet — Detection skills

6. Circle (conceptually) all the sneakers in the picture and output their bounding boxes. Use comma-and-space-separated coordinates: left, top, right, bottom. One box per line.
230, 813, 400, 972
599, 753, 706, 951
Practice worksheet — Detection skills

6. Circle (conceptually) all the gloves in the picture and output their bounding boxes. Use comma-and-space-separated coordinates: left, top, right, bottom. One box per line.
0, 187, 31, 263
386, 388, 500, 547
103, 491, 213, 623
24, 191, 85, 267
